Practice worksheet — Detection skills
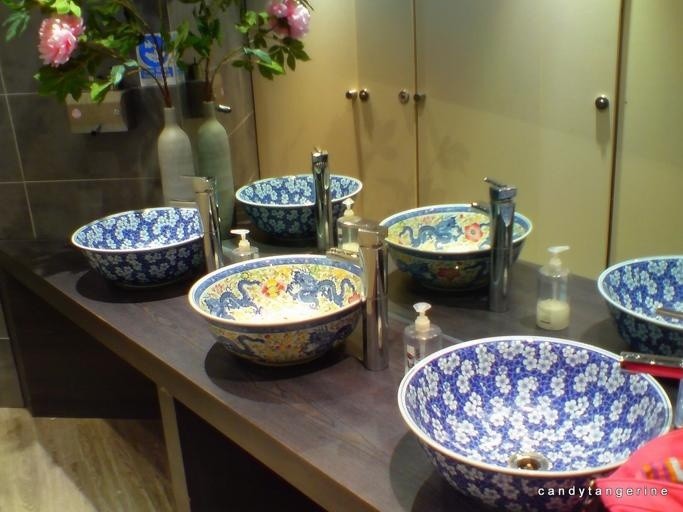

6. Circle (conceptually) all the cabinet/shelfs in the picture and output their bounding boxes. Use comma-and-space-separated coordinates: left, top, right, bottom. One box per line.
248, 1, 417, 225
412, 1, 683, 286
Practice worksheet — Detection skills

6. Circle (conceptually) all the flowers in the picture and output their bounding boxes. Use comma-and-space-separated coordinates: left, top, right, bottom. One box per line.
0, 0, 178, 112
171, 0, 314, 101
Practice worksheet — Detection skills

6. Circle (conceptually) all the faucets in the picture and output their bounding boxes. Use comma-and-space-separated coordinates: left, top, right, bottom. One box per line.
180, 173, 223, 272
656, 308, 683, 320
340, 220, 389, 371
310, 147, 333, 250
471, 177, 516, 312
619, 350, 683, 379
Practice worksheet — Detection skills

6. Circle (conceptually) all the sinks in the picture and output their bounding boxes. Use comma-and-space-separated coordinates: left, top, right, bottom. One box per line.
235, 174, 363, 241
597, 255, 683, 356
70, 207, 204, 289
378, 203, 533, 293
186, 255, 366, 368
398, 335, 674, 512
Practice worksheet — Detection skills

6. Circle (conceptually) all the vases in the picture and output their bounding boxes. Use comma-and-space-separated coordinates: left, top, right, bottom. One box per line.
194, 92, 234, 236
153, 102, 196, 212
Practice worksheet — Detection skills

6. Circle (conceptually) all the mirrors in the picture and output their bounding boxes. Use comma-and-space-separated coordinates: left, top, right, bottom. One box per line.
163, 2, 682, 431
